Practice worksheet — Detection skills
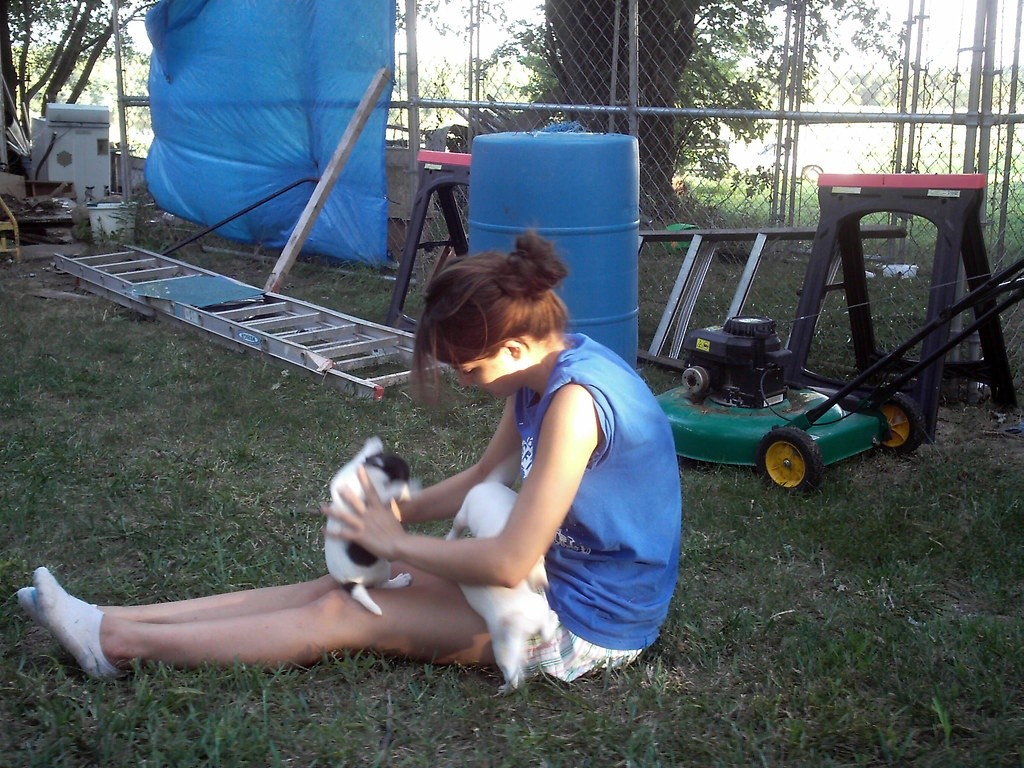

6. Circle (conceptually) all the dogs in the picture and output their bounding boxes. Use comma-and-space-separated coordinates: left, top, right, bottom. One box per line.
324, 434, 414, 615
444, 450, 561, 695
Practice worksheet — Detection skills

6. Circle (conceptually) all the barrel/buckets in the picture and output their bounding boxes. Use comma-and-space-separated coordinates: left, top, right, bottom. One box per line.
464, 129, 639, 378
881, 264, 918, 277
86, 202, 137, 245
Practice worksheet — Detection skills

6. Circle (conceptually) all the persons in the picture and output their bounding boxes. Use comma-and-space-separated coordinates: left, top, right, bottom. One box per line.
13, 232, 683, 695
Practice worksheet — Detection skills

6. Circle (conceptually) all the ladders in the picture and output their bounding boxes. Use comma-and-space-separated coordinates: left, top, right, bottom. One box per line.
420, 225, 910, 409
51, 244, 458, 403
